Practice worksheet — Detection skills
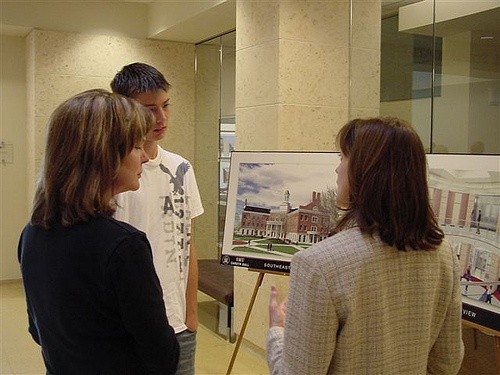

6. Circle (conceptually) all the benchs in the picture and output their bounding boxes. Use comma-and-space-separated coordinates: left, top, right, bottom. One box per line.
197, 259, 234, 342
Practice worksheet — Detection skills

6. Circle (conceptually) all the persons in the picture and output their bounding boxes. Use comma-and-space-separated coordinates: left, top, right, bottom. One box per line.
17, 88, 180, 375
485, 286, 493, 303
476, 209, 482, 235
109, 62, 204, 375
267, 117, 465, 375
